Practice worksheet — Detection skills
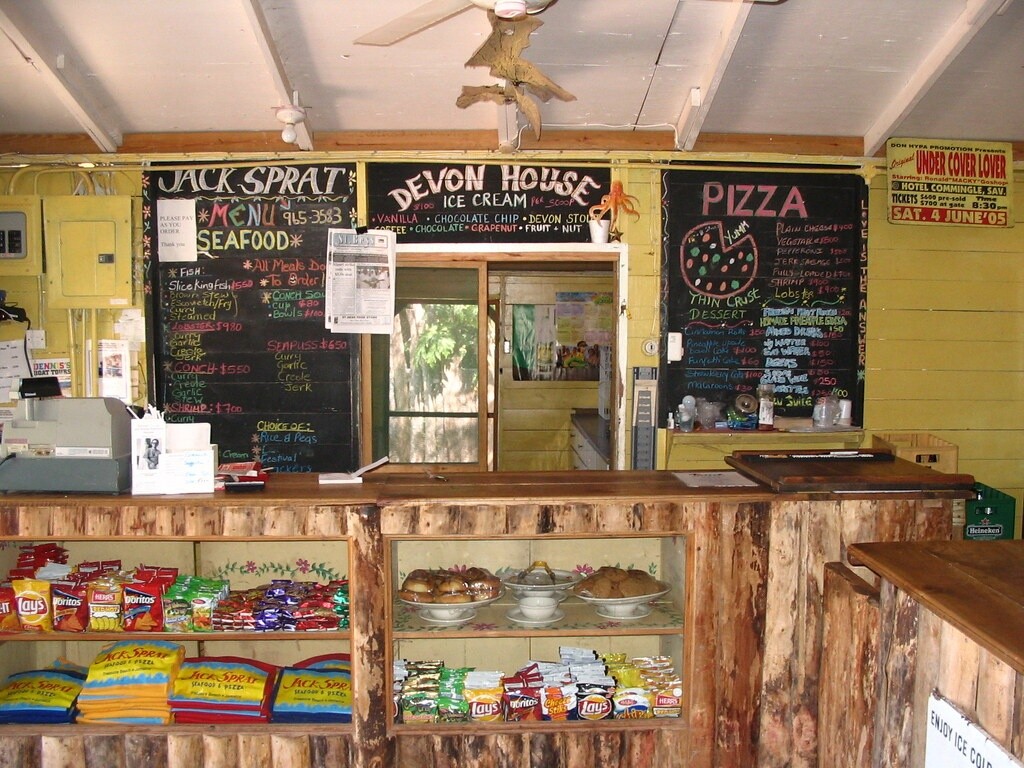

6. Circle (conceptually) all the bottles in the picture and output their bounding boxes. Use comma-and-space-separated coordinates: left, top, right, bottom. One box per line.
976, 488, 995, 515
755, 383, 775, 430
666, 412, 674, 430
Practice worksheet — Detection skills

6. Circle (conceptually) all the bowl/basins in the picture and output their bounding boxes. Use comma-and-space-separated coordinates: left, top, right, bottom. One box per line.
428, 608, 468, 619
519, 597, 558, 620
602, 603, 642, 617
522, 589, 555, 598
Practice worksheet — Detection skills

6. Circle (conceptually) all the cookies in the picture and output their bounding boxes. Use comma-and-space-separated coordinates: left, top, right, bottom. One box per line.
583, 566, 661, 598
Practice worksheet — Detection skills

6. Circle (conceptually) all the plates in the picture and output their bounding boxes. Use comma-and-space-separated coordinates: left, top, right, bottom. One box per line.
398, 569, 672, 627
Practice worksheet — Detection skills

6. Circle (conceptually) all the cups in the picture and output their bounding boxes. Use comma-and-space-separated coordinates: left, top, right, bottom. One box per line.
813, 397, 852, 430
674, 396, 715, 432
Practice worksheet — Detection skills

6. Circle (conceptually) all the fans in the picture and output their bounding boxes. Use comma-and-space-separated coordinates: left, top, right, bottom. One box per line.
352, 0, 551, 48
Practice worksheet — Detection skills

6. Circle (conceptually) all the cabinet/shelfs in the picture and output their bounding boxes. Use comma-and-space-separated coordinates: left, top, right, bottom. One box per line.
0, 469, 975, 768
571, 425, 611, 471
42, 194, 134, 309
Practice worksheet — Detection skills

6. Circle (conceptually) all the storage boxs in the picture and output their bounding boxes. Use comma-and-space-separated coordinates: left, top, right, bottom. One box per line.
871, 433, 958, 475
964, 481, 1016, 539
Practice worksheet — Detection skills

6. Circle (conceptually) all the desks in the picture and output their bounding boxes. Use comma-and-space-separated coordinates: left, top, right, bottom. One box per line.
849, 540, 1023, 767
657, 424, 864, 470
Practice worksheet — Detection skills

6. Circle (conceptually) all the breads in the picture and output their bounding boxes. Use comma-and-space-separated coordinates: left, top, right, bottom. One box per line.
397, 567, 501, 604
